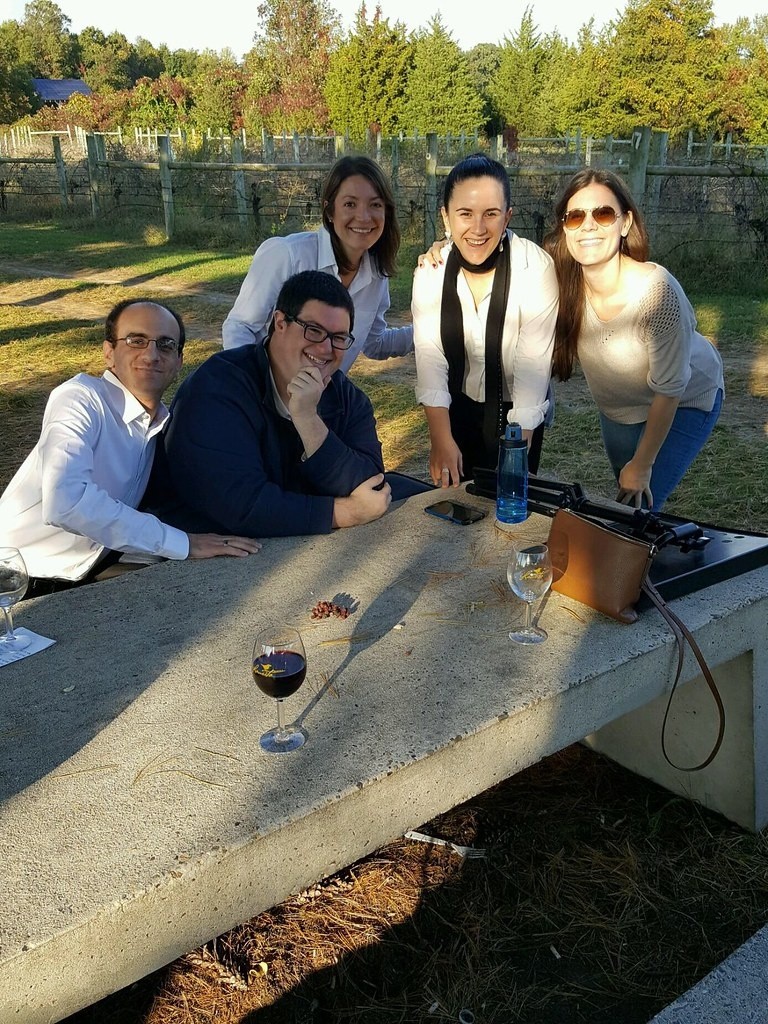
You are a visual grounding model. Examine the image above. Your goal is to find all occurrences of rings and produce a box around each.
[224,540,228,546]
[442,468,449,472]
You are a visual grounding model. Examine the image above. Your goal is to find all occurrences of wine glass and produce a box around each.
[507,540,552,645]
[0,547,32,653]
[251,627,307,752]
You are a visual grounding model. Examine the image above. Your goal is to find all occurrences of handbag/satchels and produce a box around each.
[538,507,657,625]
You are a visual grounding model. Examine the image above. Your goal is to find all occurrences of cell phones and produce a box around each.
[425,500,485,526]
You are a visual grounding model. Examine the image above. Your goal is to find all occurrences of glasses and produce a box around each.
[110,333,184,355]
[560,205,624,232]
[282,311,356,351]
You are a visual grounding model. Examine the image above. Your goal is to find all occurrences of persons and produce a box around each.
[1,296,262,605]
[220,156,414,375]
[139,268,392,535]
[411,151,559,488]
[419,168,725,511]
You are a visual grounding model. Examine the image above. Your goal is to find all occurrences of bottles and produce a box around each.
[497,422,528,523]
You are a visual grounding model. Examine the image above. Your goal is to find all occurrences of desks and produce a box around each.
[0,478,768,1024]
